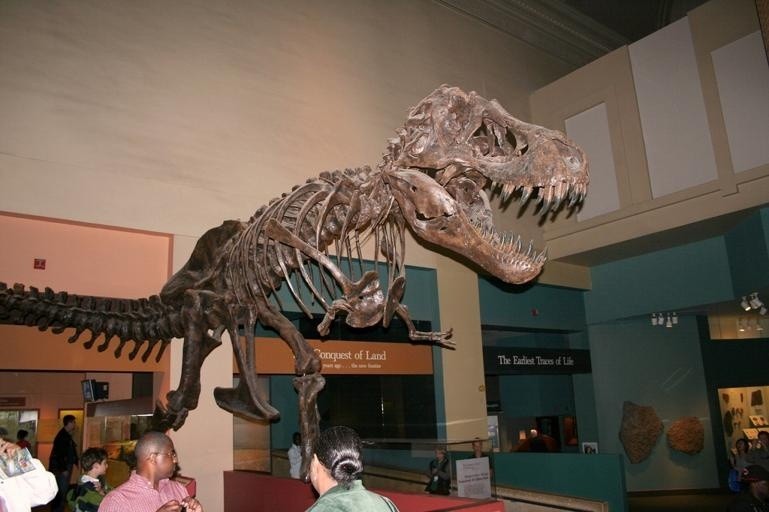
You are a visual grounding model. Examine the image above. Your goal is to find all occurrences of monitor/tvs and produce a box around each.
[81,379,97,402]
[96,382,109,401]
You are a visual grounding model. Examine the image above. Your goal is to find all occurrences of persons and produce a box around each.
[304,425,400,512]
[470,437,493,481]
[424,445,451,495]
[97,431,203,512]
[527,429,550,453]
[70,447,109,512]
[0,415,78,512]
[728,431,769,512]
[287,432,302,480]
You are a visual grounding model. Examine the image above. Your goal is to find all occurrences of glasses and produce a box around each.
[149,450,178,459]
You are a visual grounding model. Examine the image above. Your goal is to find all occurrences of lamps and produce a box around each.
[738,292,768,316]
[651,310,679,329]
